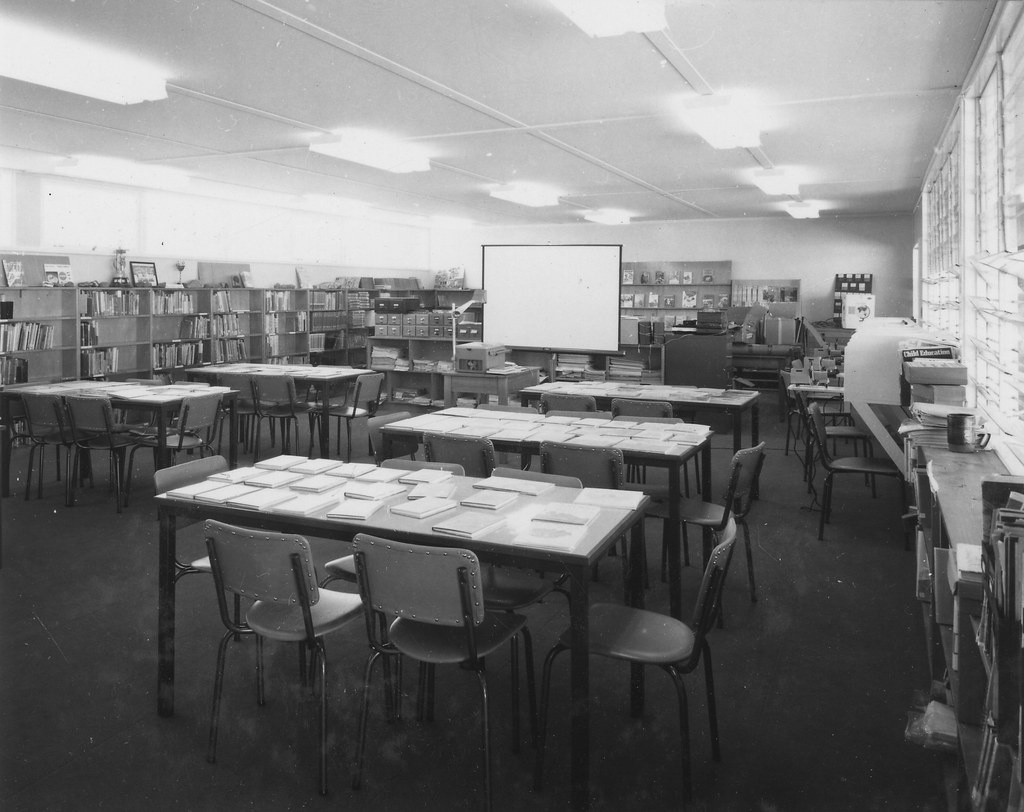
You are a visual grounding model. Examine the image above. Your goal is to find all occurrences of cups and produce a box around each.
[947,414,975,453]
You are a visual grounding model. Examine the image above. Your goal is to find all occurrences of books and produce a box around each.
[347,291,369,348]
[108,389,185,403]
[167,455,645,554]
[3,259,27,287]
[384,407,711,454]
[212,291,248,362]
[621,270,729,326]
[0,321,54,384]
[524,381,727,403]
[153,289,207,385]
[310,290,346,351]
[80,290,139,376]
[265,290,307,364]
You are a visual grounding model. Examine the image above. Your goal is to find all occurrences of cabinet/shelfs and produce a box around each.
[365,336,665,422]
[0,286,482,448]
[731,279,801,346]
[911,446,1024,812]
[619,260,731,345]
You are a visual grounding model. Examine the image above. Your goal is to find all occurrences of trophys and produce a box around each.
[109,249,132,287]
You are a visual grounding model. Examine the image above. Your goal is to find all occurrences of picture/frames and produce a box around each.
[130,260,159,287]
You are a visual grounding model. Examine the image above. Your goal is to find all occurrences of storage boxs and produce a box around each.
[767,318,796,345]
[638,322,665,370]
[904,359,967,385]
[697,311,727,334]
[912,384,965,408]
[842,293,875,330]
[375,298,483,339]
[455,341,505,372]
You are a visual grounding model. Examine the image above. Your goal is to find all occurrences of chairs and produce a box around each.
[778,348,913,551]
[0,373,764,812]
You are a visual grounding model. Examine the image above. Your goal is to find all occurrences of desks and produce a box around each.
[442,366,541,464]
[517,380,760,523]
[804,323,855,354]
[185,363,376,458]
[379,407,712,621]
[156,456,652,812]
[0,380,241,504]
